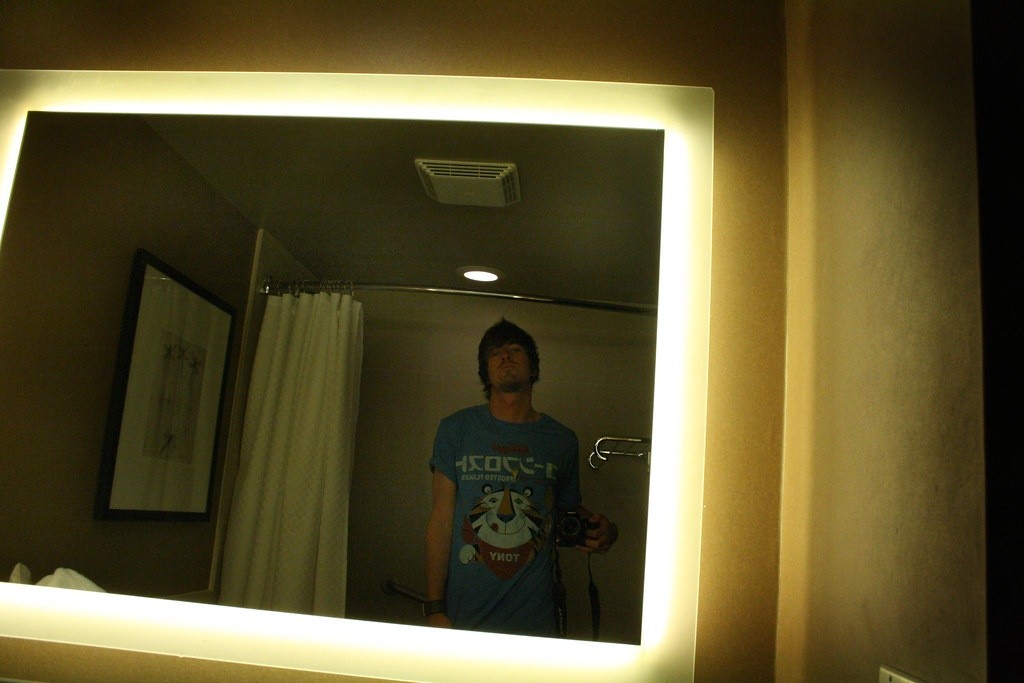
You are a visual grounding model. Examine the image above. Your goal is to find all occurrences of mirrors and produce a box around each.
[0,69,718,683]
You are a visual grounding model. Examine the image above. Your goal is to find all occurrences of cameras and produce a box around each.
[551,513,600,547]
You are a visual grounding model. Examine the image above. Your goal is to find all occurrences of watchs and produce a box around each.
[423,599,446,616]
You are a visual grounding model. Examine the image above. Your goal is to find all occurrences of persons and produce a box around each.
[426,317,621,642]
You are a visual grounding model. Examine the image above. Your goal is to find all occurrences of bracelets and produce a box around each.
[610,523,618,541]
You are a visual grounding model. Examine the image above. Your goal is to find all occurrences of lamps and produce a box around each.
[455,266,506,282]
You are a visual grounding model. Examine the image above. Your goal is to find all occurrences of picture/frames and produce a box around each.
[95,248,238,523]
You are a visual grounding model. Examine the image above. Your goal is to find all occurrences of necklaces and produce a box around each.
[488,408,535,482]
